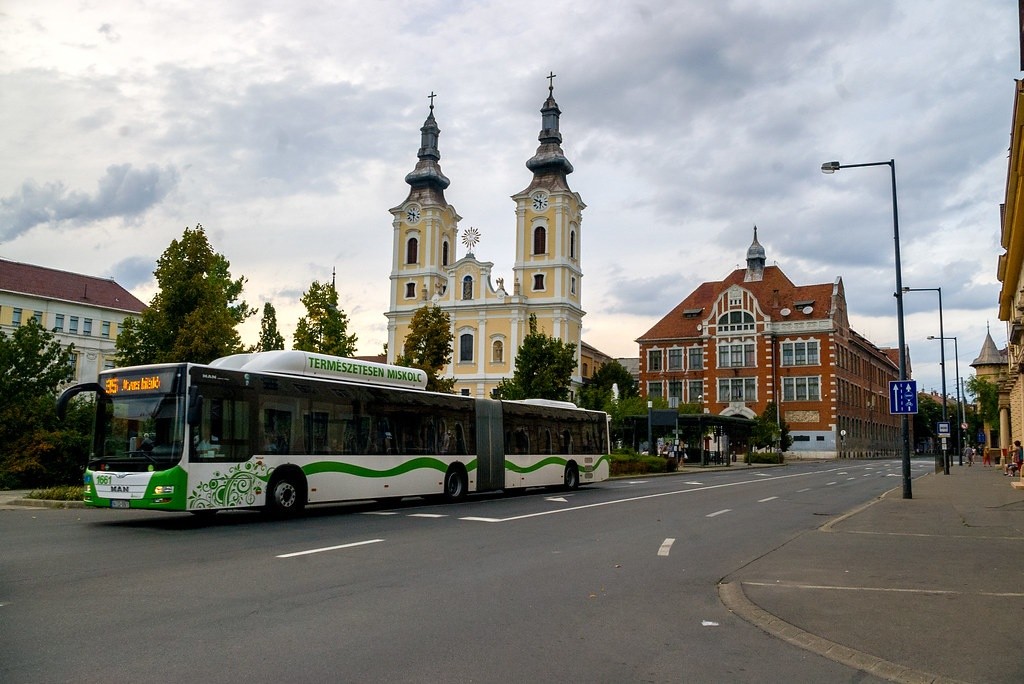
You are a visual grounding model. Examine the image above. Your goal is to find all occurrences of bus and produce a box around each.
[53,349,612,520]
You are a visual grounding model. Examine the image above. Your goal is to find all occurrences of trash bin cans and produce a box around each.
[995,456,1000,464]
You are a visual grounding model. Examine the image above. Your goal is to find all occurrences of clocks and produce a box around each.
[532,193,548,210]
[406,206,420,223]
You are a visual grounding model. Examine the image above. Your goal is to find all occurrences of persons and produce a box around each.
[193,417,221,458]
[964,444,973,466]
[1007,445,1023,476]
[971,446,977,464]
[983,442,993,467]
[1003,440,1023,477]
[140,433,152,444]
[661,438,684,469]
[256,430,343,453]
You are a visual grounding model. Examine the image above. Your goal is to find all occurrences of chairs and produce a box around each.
[704,450,729,464]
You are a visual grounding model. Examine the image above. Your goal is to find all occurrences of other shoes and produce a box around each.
[1003,474,1008,476]
[1012,474,1015,476]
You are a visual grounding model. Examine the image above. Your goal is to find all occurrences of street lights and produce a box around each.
[817,158,912,499]
[903,287,950,475]
[927,335,962,467]
